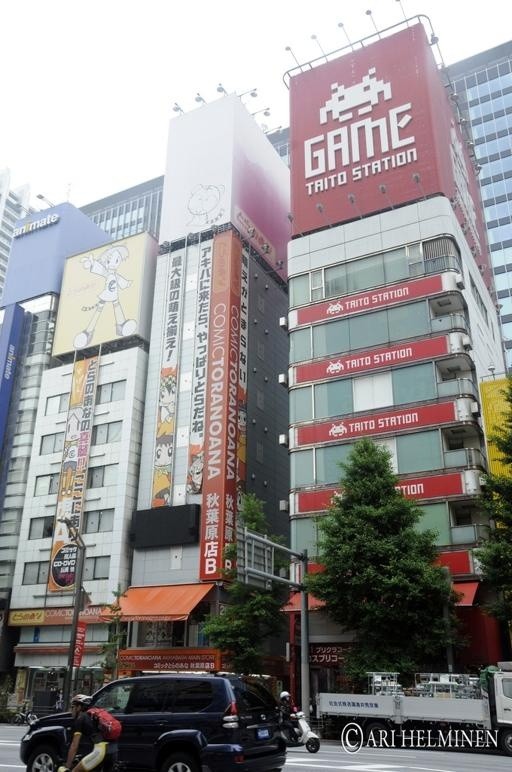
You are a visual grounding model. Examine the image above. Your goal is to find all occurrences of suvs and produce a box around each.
[19,669,289,772]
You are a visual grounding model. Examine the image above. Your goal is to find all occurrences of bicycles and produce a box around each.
[10,700,38,727]
[55,755,131,772]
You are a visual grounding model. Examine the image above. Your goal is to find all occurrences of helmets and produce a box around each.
[72,693,94,706]
[279,691,292,700]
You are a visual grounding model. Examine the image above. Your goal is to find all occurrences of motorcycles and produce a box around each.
[278,710,322,754]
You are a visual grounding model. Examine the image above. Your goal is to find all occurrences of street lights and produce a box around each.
[54,515,88,714]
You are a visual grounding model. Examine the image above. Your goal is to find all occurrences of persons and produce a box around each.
[276,691,299,743]
[58,693,123,770]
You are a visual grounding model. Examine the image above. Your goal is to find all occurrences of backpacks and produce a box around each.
[85,705,123,741]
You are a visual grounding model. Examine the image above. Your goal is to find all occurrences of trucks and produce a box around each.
[314,660,512,759]
